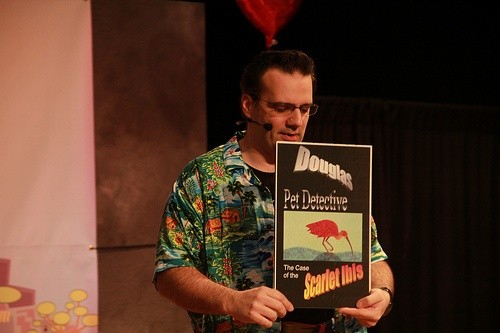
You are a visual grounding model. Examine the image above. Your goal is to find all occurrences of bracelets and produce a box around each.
[380,287,393,317]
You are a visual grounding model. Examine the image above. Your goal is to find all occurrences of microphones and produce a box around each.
[245,118,273,131]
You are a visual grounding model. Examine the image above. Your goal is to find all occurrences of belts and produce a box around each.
[281,323,336,333]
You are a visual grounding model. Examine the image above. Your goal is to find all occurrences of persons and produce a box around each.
[152,50,394,333]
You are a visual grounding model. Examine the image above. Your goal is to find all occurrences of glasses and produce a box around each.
[256,97,319,119]
